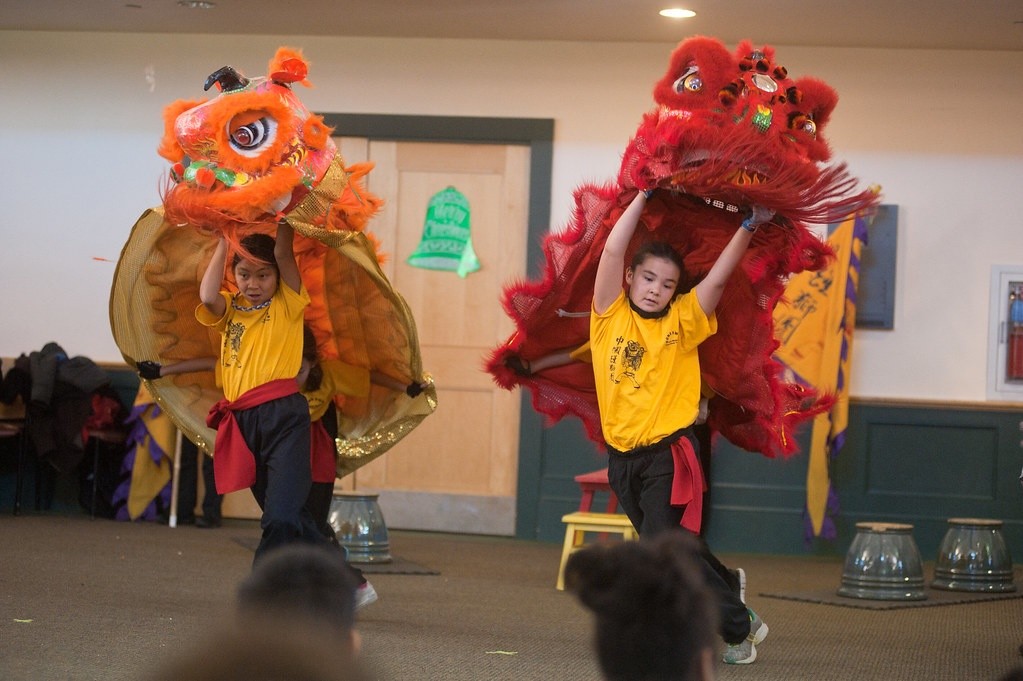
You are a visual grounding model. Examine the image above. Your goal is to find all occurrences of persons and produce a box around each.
[505,338,714,542]
[135,325,425,561]
[563,542,716,681]
[591,189,776,666]
[195,217,378,626]
[238,545,360,681]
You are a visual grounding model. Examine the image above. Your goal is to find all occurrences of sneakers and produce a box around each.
[722,608,768,666]
[728,567,747,604]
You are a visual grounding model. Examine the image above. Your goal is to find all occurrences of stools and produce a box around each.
[573,468,619,545]
[556,511,640,591]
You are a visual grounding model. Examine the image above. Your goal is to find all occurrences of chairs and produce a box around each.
[85,405,135,520]
[0,395,29,517]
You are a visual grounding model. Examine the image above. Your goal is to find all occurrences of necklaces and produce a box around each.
[232,291,272,312]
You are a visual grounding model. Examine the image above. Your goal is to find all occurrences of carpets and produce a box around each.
[231,535,441,576]
[759,582,1023,611]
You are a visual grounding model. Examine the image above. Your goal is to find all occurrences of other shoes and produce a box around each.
[197,514,225,526]
[164,510,195,523]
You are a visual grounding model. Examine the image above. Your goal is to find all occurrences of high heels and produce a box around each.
[351,581,378,610]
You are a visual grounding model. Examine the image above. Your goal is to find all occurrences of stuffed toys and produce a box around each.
[484,36,877,460]
[108,46,439,478]
